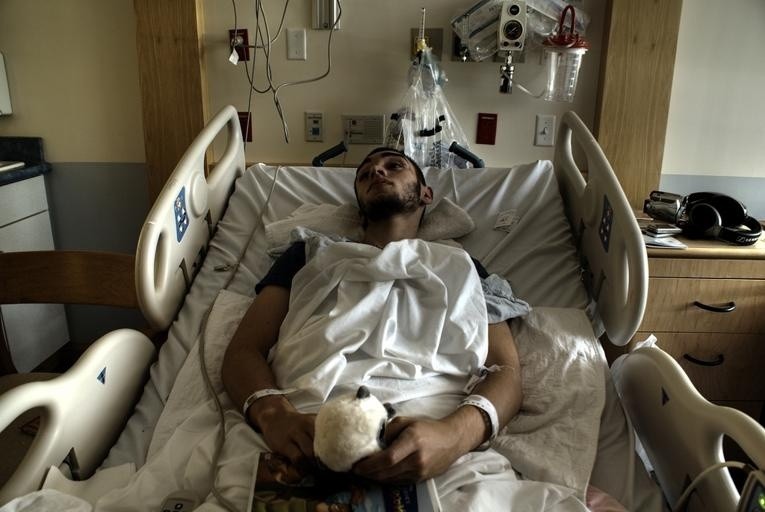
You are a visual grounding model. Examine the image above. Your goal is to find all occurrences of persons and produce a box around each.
[217,146,524,489]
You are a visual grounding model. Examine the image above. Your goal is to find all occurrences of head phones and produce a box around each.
[682,191,763,247]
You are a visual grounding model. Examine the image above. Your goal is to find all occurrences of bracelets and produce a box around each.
[457,393,500,453]
[240,389,288,415]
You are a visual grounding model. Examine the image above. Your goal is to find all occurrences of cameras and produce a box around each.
[643,190,685,227]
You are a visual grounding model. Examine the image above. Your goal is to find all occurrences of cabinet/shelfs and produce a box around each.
[625,210,765,404]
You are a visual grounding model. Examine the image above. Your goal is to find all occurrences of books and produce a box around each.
[245,450,443,511]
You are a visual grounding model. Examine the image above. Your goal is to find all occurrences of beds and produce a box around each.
[0,110,765,512]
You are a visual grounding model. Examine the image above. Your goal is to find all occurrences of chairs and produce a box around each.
[1,249,158,506]
